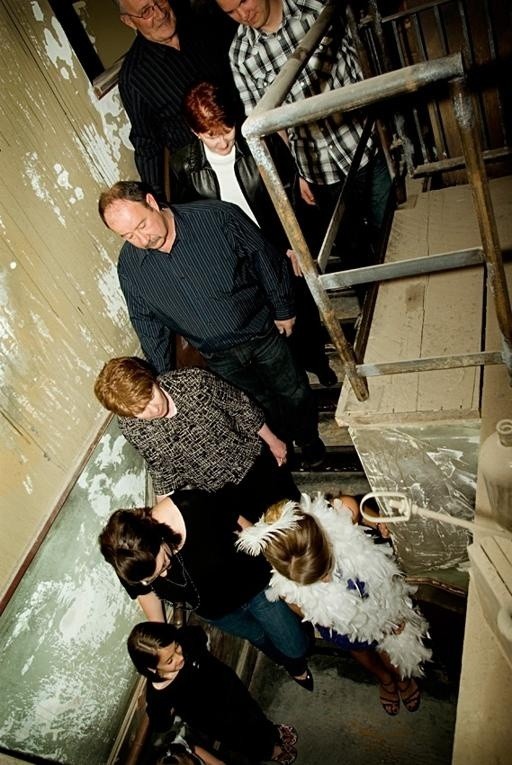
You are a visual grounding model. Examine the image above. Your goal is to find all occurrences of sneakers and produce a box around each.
[303,439,328,466]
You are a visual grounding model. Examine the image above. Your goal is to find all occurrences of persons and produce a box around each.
[127,621,299,765]
[174,83,340,386]
[234,492,450,717]
[99,486,347,692]
[117,0,235,205]
[144,741,222,765]
[94,357,301,525]
[98,180,327,474]
[219,0,396,316]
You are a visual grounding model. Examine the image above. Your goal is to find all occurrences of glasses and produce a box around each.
[139,545,172,585]
[128,0,166,19]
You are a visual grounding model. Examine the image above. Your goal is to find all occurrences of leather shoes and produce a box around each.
[270,724,297,764]
[316,366,337,387]
[288,665,313,692]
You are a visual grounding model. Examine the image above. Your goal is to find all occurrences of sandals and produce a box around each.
[379,675,420,715]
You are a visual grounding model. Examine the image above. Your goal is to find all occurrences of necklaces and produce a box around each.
[149,552,201,615]
